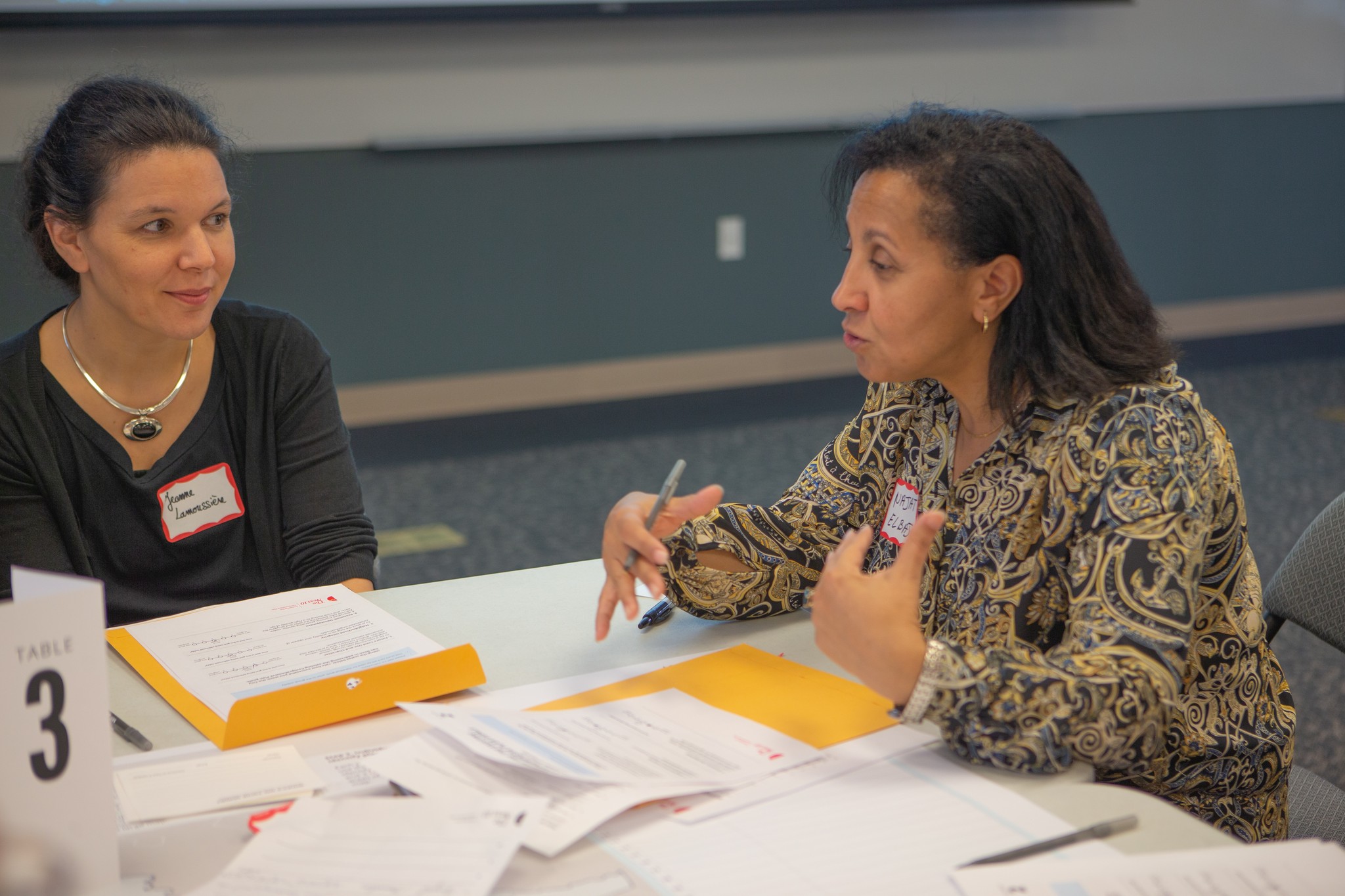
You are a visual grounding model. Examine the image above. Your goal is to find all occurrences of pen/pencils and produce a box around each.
[957,815,1142,868]
[637,596,675,630]
[622,460,686,570]
[109,713,153,752]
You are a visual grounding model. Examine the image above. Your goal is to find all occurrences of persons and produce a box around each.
[0,78,378,632]
[594,103,1294,846]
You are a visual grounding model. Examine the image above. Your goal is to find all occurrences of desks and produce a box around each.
[108,551,1248,896]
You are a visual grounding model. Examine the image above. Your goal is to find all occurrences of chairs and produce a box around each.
[1262,485,1345,849]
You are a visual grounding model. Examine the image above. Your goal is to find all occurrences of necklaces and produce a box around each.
[62,297,193,440]
[960,390,1032,438]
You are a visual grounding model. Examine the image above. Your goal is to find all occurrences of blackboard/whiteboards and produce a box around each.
[1,4,1343,431]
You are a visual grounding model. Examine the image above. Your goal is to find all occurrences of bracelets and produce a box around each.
[888,637,943,728]
